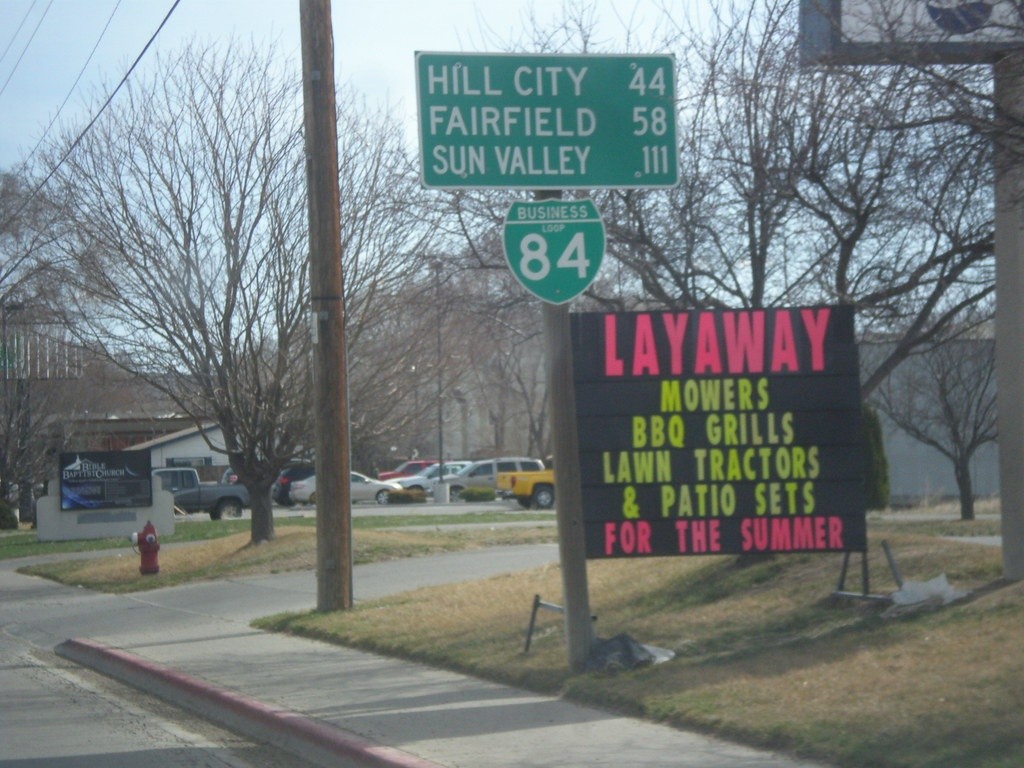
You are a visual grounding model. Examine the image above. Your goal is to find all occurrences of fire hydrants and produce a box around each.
[125,520,163,576]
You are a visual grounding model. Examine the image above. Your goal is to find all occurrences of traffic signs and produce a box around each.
[412,49,679,190]
[502,197,606,308]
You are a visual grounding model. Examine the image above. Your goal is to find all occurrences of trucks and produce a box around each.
[494,468,557,509]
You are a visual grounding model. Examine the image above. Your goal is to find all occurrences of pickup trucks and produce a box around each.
[432,456,545,505]
[151,467,251,522]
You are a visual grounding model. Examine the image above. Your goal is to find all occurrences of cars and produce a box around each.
[288,468,404,508]
[384,459,474,500]
[269,461,316,507]
[378,459,441,480]
